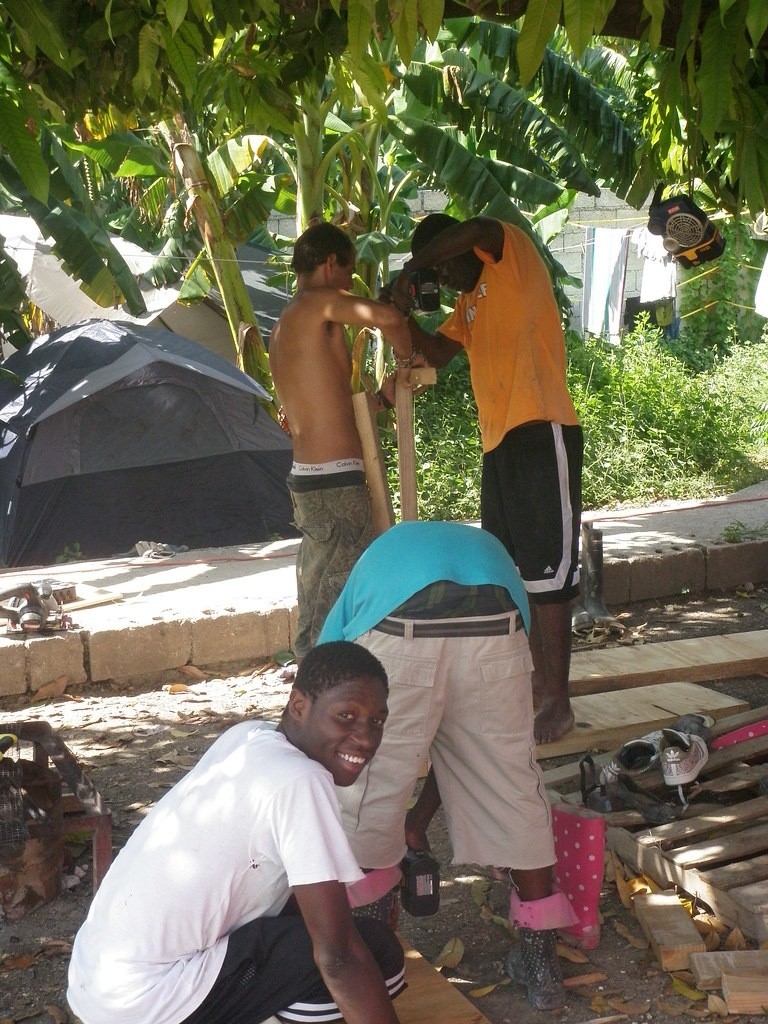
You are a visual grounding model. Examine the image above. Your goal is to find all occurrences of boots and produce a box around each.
[546,787,607,950]
[345,865,405,933]
[505,885,578,1009]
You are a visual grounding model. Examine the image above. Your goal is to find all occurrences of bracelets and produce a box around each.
[389,346,416,368]
[377,390,392,410]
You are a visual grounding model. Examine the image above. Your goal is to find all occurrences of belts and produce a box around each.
[374,615,524,637]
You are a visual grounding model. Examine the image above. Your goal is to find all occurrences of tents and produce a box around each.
[0,215,293,571]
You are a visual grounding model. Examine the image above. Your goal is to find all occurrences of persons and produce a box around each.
[267,223,416,667]
[66,642,408,1024]
[314,522,566,1011]
[380,214,585,745]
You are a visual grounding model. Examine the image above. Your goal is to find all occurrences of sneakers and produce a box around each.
[613,728,709,789]
